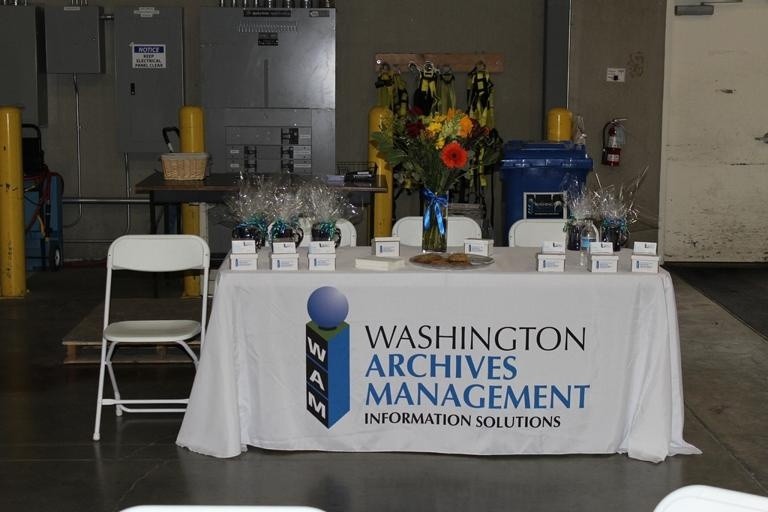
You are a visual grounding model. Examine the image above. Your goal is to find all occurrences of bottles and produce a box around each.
[567,225,582,250]
[580,218,599,268]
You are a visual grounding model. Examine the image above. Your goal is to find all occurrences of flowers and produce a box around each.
[372,113,504,250]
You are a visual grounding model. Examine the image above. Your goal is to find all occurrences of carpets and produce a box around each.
[676,267,768,341]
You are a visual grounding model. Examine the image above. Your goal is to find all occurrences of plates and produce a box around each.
[408,252,494,270]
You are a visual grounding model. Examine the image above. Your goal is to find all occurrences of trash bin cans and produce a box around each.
[499,140,593,247]
[18,174,65,273]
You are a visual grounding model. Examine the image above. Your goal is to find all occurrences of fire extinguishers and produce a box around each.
[602,118,628,168]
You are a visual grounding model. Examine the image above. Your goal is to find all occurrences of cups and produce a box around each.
[602,227,630,251]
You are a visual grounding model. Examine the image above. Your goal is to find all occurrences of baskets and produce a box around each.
[161,152,209,180]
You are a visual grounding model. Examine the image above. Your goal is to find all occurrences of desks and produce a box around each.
[177,246,702,465]
[134,173,388,296]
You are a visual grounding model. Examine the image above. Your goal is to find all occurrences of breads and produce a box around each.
[449,253,468,262]
[416,254,441,264]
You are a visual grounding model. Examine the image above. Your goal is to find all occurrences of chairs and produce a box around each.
[509,218,568,248]
[92,235,211,442]
[391,217,482,246]
[265,217,357,247]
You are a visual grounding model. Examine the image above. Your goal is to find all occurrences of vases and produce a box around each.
[423,181,448,253]
